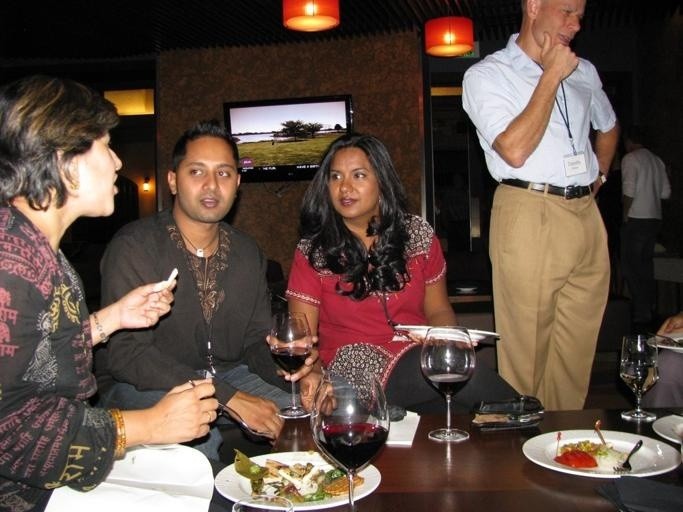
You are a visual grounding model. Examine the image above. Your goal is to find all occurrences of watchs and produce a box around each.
[596,170,607,187]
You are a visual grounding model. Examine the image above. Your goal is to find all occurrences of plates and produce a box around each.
[214,450,381,511]
[652,414,683,444]
[521,429,682,478]
[647,333,682,353]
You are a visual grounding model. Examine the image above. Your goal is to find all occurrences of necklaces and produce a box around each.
[177,226,218,256]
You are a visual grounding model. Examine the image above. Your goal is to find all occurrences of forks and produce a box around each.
[614,438,643,473]
[189,379,275,438]
[655,334,683,347]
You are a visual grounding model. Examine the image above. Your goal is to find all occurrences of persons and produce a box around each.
[0,77,218,511]
[462,0,617,411]
[657,310,683,341]
[285,134,524,414]
[620,126,670,333]
[94,123,318,443]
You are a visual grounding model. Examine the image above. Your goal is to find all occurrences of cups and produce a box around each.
[232,494,294,511]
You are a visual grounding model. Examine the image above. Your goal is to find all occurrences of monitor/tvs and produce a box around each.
[220,93,353,184]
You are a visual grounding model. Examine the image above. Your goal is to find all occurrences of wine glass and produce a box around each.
[309,372,391,512]
[419,326,476,443]
[269,312,313,419]
[620,334,660,422]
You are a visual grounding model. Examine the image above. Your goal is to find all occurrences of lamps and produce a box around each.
[281,0,340,33]
[143,176,149,191]
[423,7,474,58]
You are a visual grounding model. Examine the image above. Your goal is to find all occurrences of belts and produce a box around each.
[501,179,595,200]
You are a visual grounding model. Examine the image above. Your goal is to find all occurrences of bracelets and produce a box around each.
[111,408,125,459]
[92,312,107,344]
